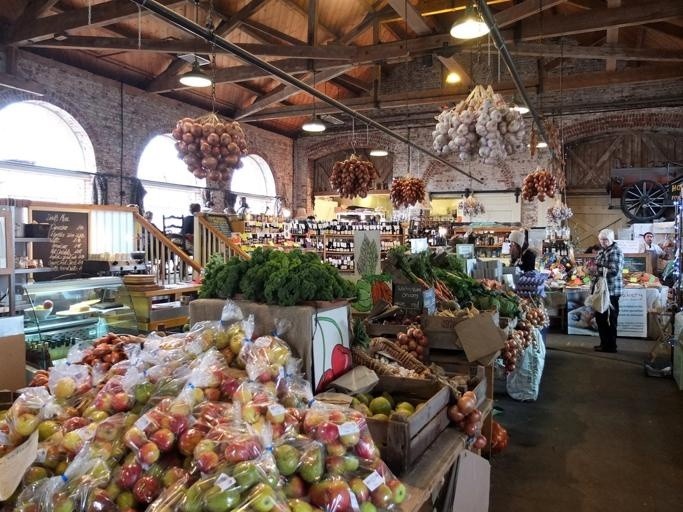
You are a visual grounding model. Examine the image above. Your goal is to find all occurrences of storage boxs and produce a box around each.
[313,303,511,472]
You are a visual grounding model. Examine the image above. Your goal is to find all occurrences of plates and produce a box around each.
[123,274,155,284]
[55,308,99,316]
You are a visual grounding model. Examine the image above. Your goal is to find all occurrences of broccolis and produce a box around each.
[196,246,359,307]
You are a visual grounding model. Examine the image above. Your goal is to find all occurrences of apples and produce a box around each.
[44,302,52,308]
[0,326,425,512]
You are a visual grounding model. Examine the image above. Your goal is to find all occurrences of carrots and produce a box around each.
[370,279,394,309]
[416,277,454,305]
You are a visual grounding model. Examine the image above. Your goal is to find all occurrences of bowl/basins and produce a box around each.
[23,309,51,319]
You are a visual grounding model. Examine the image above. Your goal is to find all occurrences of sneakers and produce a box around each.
[594,344,618,354]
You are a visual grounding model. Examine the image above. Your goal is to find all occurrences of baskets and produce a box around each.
[353,336,427,378]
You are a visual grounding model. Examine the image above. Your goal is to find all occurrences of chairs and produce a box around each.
[158,214,188,281]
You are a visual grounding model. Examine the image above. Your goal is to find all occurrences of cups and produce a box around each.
[179,296,190,305]
[18,256,43,269]
[103,253,130,261]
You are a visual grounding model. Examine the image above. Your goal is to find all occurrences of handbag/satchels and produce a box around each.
[590,277,611,313]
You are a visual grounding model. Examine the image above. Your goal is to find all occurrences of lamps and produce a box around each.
[300,67,326,135]
[450,0,490,41]
[176,0,211,88]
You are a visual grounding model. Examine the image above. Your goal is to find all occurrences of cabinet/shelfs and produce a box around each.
[10,278,143,386]
[229,216,654,276]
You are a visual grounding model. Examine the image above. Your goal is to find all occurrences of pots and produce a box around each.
[23,224,50,238]
[130,252,146,259]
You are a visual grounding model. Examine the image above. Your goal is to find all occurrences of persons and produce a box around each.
[509,229,535,269]
[641,233,663,256]
[588,229,623,352]
[584,244,602,254]
[181,203,201,256]
[143,212,161,232]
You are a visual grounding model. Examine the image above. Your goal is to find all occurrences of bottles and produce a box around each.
[474,233,509,258]
[25,333,77,370]
[542,233,567,256]
[244,214,402,274]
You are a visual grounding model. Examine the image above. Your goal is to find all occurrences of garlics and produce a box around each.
[458,196,485,217]
[546,203,571,225]
[432,85,526,160]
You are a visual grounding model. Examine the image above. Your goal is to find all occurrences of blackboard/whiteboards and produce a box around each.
[204,213,232,244]
[26,205,92,282]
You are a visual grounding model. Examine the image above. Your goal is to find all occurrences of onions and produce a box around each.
[390,174,425,207]
[500,309,545,372]
[329,159,377,199]
[519,171,558,202]
[171,118,249,181]
[448,390,509,453]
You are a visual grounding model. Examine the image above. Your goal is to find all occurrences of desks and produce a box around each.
[562,282,663,337]
